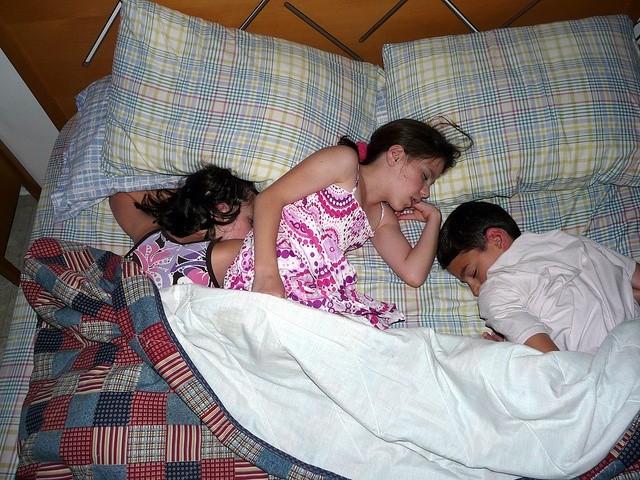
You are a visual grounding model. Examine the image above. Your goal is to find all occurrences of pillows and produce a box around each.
[382,15,639,207]
[52,75,260,226]
[101,1,378,194]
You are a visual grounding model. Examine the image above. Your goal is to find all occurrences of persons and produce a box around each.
[109,162,259,293]
[222,113,474,331]
[435,202,639,354]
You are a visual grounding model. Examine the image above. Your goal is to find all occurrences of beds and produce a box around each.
[1,1,640,479]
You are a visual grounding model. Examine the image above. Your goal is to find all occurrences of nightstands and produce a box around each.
[0,139,44,288]
